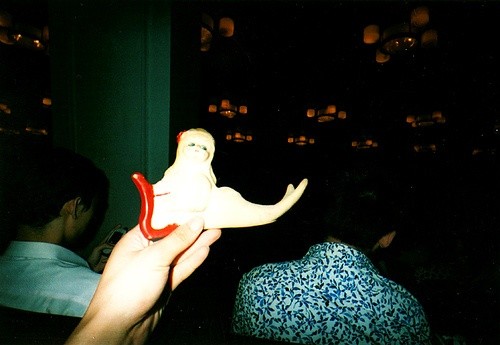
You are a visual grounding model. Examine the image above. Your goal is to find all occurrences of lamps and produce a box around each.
[198,5,446,150]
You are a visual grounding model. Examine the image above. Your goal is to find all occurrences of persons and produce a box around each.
[131,127,309,242]
[64,218,221,345]
[230,147,431,345]
[1,149,111,318]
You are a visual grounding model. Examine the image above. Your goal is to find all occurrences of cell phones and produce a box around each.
[102,228,127,255]
